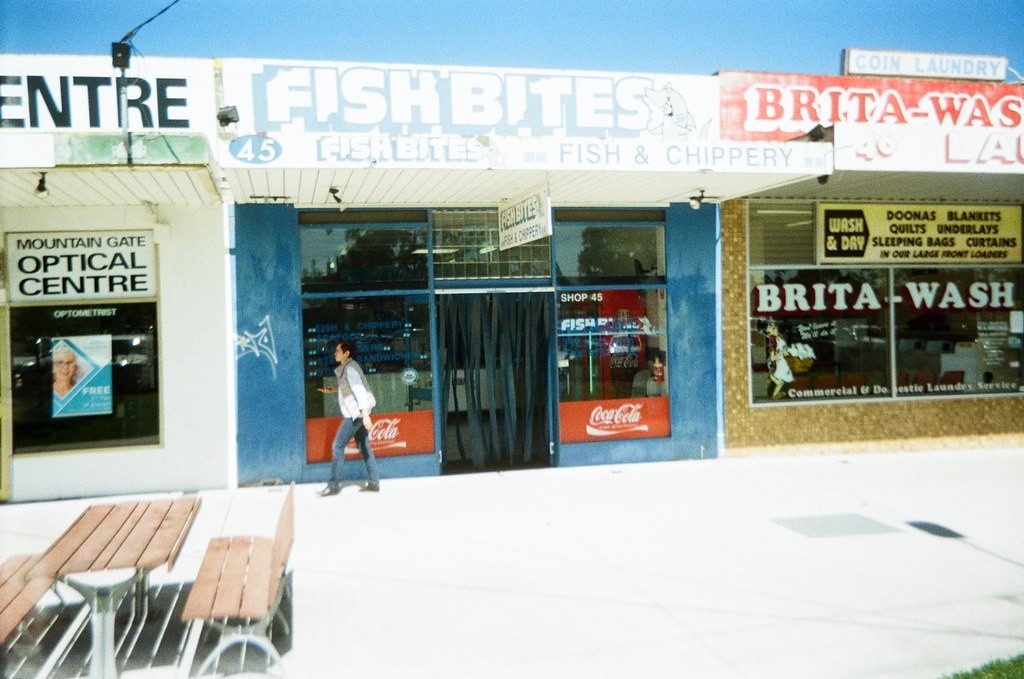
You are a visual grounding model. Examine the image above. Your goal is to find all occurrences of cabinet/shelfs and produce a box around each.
[846,343,976,384]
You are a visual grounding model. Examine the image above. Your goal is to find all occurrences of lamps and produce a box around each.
[217,105,240,128]
[35,172,49,198]
[327,188,349,213]
[689,190,706,210]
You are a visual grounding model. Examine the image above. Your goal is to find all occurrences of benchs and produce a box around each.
[184,480,296,678]
[0,553,68,647]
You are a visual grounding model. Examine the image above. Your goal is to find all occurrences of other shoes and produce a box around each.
[365,481,379,491]
[320,485,337,496]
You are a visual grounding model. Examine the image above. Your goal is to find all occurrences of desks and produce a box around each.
[22,495,202,679]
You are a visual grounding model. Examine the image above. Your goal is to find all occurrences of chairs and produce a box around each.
[788,370,966,398]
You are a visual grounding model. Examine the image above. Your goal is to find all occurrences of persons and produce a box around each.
[53,346,77,398]
[314,341,380,498]
